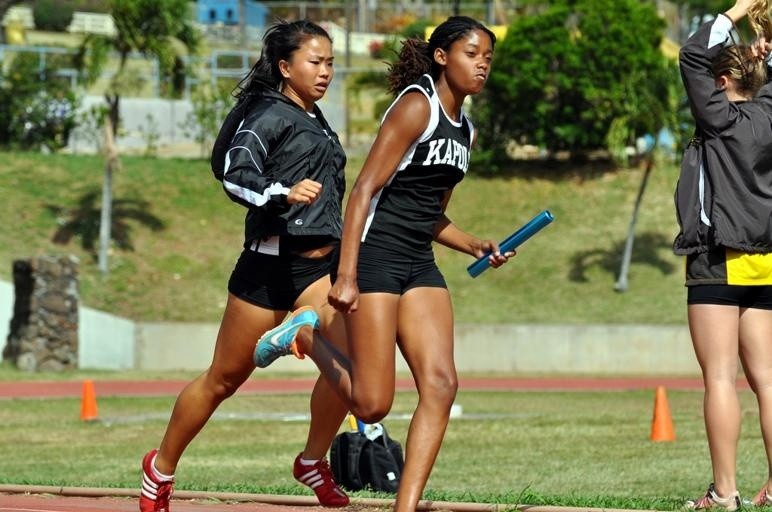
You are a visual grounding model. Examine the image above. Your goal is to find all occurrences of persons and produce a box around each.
[251,15,516,510]
[136,18,350,511]
[671,1,771,511]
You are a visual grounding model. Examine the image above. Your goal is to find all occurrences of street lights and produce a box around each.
[649,385,677,443]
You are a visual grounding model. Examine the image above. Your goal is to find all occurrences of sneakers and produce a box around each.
[293,448,350,507]
[138,448,173,512]
[253,303,320,369]
[741,486,772,508]
[684,484,741,511]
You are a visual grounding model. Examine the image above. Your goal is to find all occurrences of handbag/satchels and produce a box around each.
[329,424,407,494]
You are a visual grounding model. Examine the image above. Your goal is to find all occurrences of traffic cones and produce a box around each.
[80,382,99,420]
